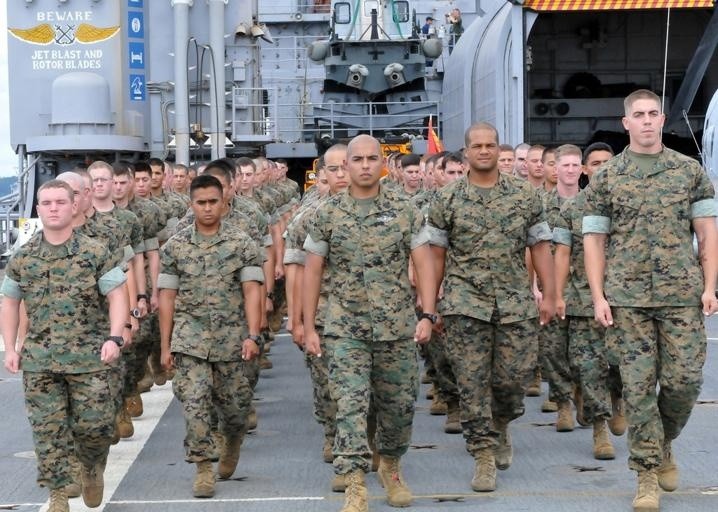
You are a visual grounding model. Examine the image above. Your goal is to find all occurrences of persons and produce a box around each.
[445,8,462,57]
[421,17,435,67]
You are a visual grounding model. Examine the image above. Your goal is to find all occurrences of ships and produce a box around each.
[8,0,717,511]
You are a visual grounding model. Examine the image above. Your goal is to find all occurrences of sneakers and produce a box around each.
[331,473,348,493]
[64,475,83,498]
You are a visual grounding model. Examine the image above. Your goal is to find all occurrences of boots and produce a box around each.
[219,301,290,480]
[189,461,219,500]
[471,447,498,493]
[338,468,371,512]
[632,469,663,512]
[78,462,107,508]
[106,340,173,446]
[656,437,681,493]
[421,371,626,470]
[45,484,71,512]
[375,453,415,508]
[321,433,338,462]
